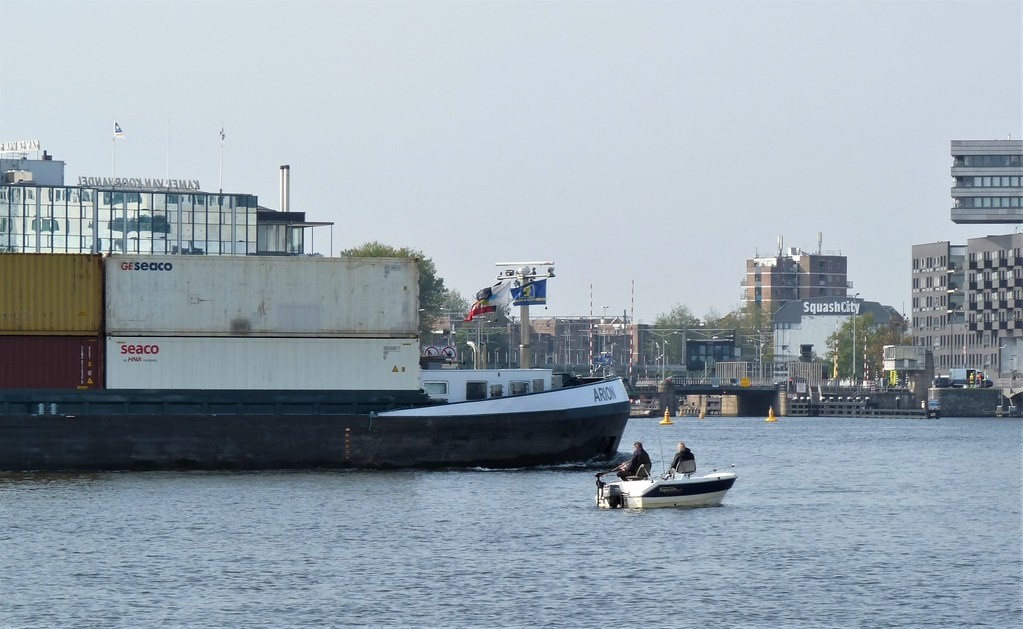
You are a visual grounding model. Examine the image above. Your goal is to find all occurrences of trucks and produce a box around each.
[948,368,993,388]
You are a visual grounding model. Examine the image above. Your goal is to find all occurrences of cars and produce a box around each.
[934,376,949,388]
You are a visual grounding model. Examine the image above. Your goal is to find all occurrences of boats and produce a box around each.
[420,368,555,403]
[595,472,737,509]
[1,153,631,476]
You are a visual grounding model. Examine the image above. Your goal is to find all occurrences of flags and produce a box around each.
[115,123,125,138]
[463,300,496,321]
[510,278,546,306]
[473,280,510,305]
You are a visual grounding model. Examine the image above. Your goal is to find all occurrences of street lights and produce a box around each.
[477,316,486,369]
[786,347,792,392]
[600,304,609,357]
[480,320,492,368]
[745,335,774,380]
[560,319,571,364]
[655,341,661,373]
[664,339,670,369]
[674,331,684,365]
[688,328,729,384]
[836,293,860,377]
[642,328,684,381]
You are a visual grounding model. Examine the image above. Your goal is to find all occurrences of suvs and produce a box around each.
[774,377,806,392]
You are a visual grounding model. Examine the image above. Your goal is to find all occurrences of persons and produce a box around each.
[616,442,651,482]
[671,442,694,477]
[977,373,981,383]
[970,373,974,384]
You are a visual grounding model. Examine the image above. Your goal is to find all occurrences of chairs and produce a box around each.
[625,462,651,481]
[676,457,696,478]
